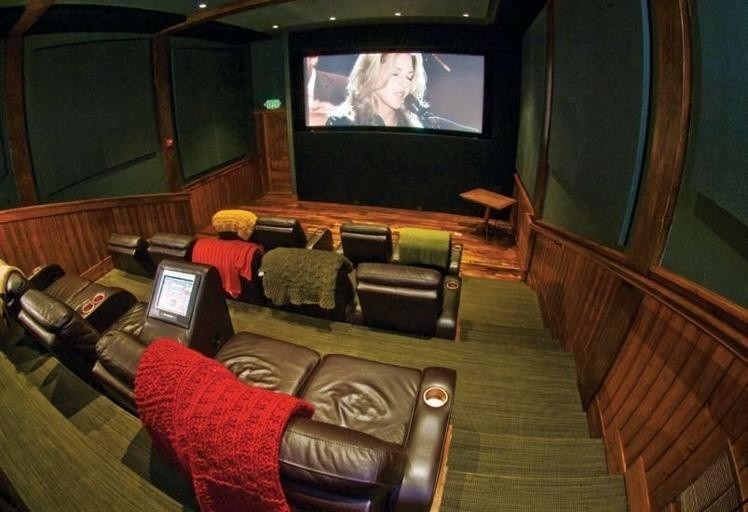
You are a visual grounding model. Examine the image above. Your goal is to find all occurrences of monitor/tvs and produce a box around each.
[149,266,200,329]
[298,49,491,140]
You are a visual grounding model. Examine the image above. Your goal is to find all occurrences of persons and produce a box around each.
[325,53,428,129]
[305,56,349,115]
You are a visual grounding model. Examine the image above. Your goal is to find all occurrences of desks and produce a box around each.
[460,188,518,242]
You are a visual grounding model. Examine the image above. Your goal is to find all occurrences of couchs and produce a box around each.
[219,353,457,511]
[0,259,67,328]
[94,328,322,441]
[188,233,262,306]
[212,210,256,242]
[106,233,155,278]
[335,223,393,267]
[147,232,193,270]
[258,248,358,324]
[355,262,463,341]
[255,217,334,252]
[17,274,148,371]
[391,228,462,276]
